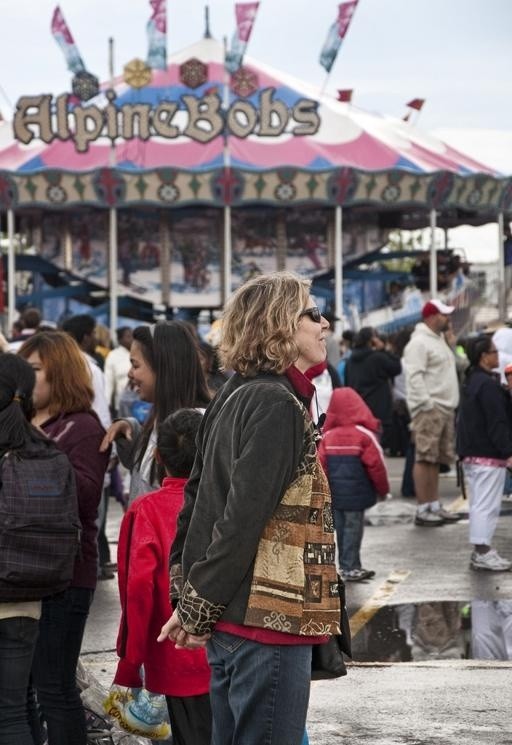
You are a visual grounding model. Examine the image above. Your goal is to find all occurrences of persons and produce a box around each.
[108,407,211,745]
[311,291,511,581]
[17,331,113,745]
[469,601,512,661]
[394,603,466,661]
[0,352,82,745]
[1,292,43,357]
[155,273,352,745]
[54,312,231,510]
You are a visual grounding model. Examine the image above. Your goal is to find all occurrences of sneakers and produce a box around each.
[339,567,375,582]
[97,559,119,580]
[470,547,512,571]
[415,505,461,527]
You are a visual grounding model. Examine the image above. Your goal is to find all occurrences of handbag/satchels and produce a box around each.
[311,578,353,681]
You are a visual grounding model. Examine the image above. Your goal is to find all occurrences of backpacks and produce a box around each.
[1,442,82,602]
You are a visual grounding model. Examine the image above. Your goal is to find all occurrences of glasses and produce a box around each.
[299,307,321,323]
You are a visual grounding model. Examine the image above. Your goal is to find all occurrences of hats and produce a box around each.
[421,299,456,319]
